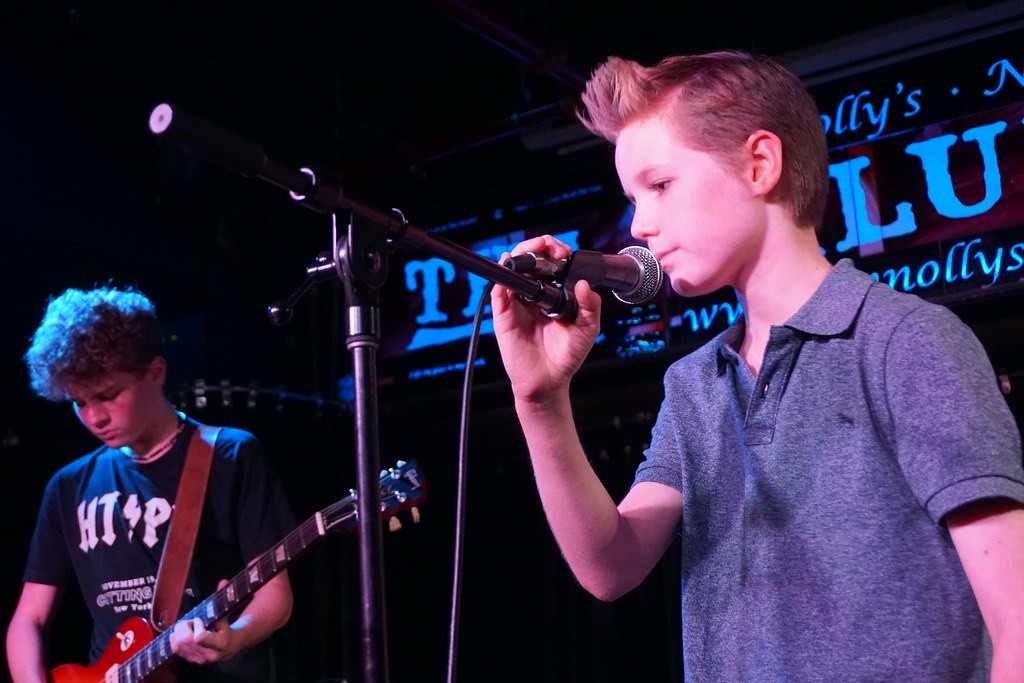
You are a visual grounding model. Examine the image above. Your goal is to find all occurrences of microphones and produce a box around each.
[507,245,664,306]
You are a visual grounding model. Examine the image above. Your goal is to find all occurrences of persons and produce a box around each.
[5,289,295,683]
[489,52,1024,683]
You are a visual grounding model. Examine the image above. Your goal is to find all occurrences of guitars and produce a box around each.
[44,454,429,683]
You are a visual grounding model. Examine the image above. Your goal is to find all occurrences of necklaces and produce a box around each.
[130,422,186,461]
